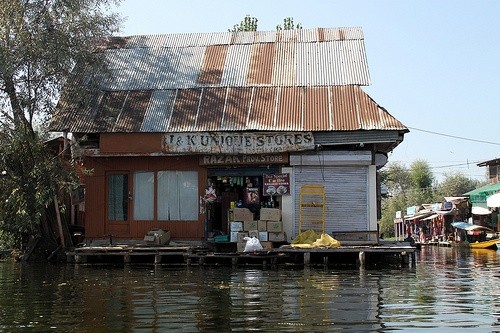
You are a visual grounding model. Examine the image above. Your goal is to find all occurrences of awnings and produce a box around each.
[463,182,500,215]
[404,207,461,221]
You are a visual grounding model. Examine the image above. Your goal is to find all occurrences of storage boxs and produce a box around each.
[258,220,266,232]
[260,207,282,221]
[268,232,285,242]
[231,221,243,232]
[233,208,254,221]
[237,243,247,252]
[259,232,268,241]
[231,232,239,242]
[144,229,172,246]
[261,242,272,248]
[266,221,282,232]
[244,220,257,231]
[249,230,258,240]
[237,232,248,243]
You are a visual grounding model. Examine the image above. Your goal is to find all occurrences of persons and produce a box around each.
[404,223,451,242]
[379,233,384,238]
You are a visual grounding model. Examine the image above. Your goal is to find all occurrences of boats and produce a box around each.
[413,222,500,248]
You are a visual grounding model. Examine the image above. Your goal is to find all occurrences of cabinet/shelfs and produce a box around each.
[299,184,326,236]
